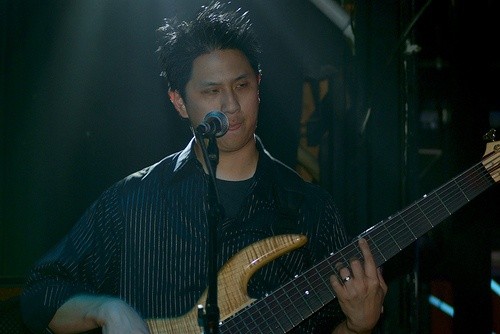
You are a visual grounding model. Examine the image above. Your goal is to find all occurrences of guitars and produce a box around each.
[143,128,500,334]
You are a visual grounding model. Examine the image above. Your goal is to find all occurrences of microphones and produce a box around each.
[195,111,229,137]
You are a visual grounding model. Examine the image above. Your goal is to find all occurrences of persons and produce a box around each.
[14,0,388,334]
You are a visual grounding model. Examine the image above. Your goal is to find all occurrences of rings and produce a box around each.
[343,276,352,284]
